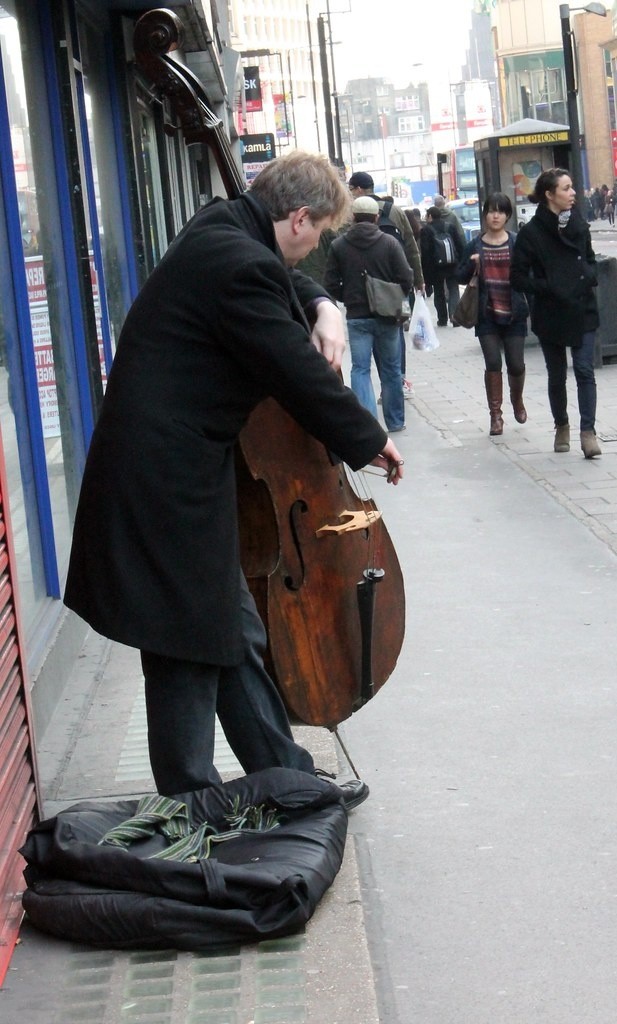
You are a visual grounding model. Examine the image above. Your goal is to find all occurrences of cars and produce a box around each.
[444,197,484,242]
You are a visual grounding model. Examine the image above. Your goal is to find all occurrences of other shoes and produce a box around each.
[389,425,406,433]
[450,318,453,323]
[437,321,447,327]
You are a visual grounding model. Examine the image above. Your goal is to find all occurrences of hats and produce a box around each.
[351,196,379,215]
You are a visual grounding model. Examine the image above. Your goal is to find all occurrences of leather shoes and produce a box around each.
[314,768,370,812]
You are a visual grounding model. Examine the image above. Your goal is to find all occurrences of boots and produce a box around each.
[508,364,527,424]
[484,370,504,435]
[580,428,602,459]
[554,424,570,452]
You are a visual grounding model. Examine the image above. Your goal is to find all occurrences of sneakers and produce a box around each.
[402,382,416,399]
[377,398,383,405]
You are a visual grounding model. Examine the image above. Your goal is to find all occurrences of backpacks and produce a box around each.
[377,200,415,270]
[425,222,461,272]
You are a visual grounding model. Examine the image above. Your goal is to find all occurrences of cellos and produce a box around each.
[130,6,409,779]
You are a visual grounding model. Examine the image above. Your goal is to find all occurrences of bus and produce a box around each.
[436,146,478,202]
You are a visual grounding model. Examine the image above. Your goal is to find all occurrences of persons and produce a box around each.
[322,168,617,458]
[63,152,407,810]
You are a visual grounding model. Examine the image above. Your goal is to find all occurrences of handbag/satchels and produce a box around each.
[365,275,412,327]
[603,203,614,214]
[453,234,480,328]
